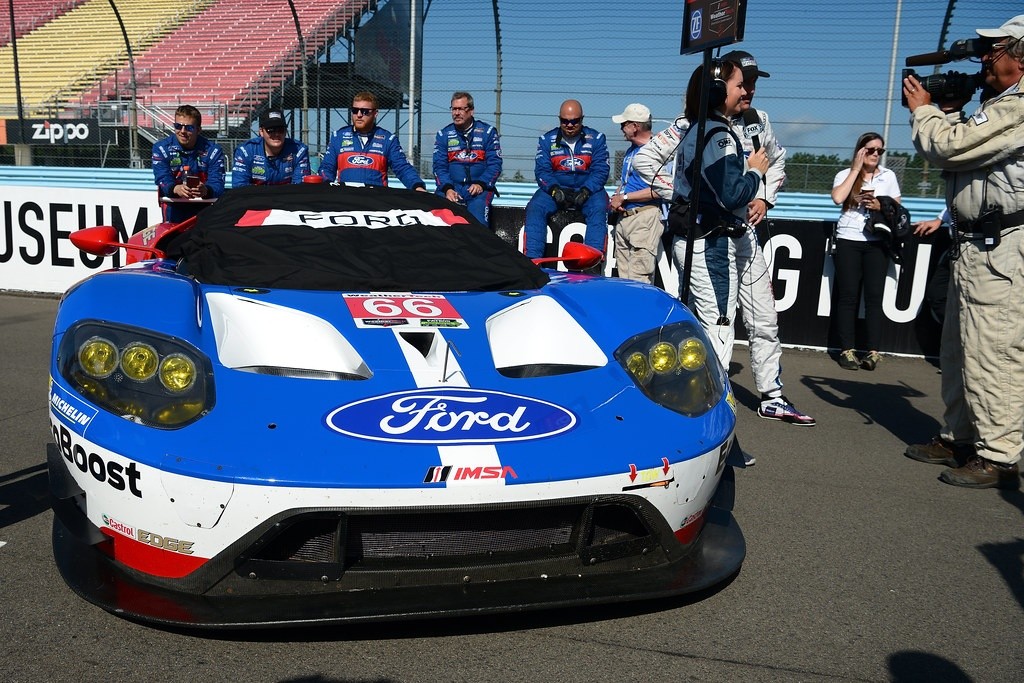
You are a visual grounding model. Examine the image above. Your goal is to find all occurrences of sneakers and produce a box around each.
[839,348,860,370]
[757,400,816,427]
[940,454,1021,491]
[861,350,883,371]
[906,433,972,469]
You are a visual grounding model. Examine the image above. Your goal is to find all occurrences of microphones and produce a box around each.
[742,108,766,184]
[905,50,955,67]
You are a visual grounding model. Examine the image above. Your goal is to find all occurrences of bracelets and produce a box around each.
[851,167,860,171]
[623,193,628,201]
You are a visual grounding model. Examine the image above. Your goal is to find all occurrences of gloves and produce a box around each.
[574,187,591,206]
[549,184,565,205]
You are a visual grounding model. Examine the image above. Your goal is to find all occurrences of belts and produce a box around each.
[958,209,1024,233]
[619,204,659,217]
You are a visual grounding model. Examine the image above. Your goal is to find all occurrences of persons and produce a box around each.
[432,91,503,229]
[607,103,666,286]
[831,132,902,370]
[903,14,1024,489]
[317,91,428,193]
[632,49,816,427]
[523,99,610,274]
[910,207,954,325]
[232,108,311,188]
[151,105,226,224]
[667,57,770,377]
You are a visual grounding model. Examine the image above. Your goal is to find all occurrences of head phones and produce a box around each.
[709,56,728,106]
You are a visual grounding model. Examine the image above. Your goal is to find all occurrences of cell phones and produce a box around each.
[186,176,199,192]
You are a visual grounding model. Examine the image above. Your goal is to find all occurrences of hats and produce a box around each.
[722,50,771,78]
[975,14,1024,41]
[612,103,651,124]
[260,107,285,128]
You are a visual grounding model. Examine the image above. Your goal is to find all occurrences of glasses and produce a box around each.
[266,127,285,133]
[558,116,584,124]
[975,41,1010,57]
[173,123,200,131]
[866,147,885,155]
[351,107,376,115]
[449,106,472,112]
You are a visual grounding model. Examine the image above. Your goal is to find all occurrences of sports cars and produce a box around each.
[46,171,750,630]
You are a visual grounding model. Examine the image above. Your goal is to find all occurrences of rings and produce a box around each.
[756,212,759,215]
[911,88,915,92]
[763,152,768,157]
